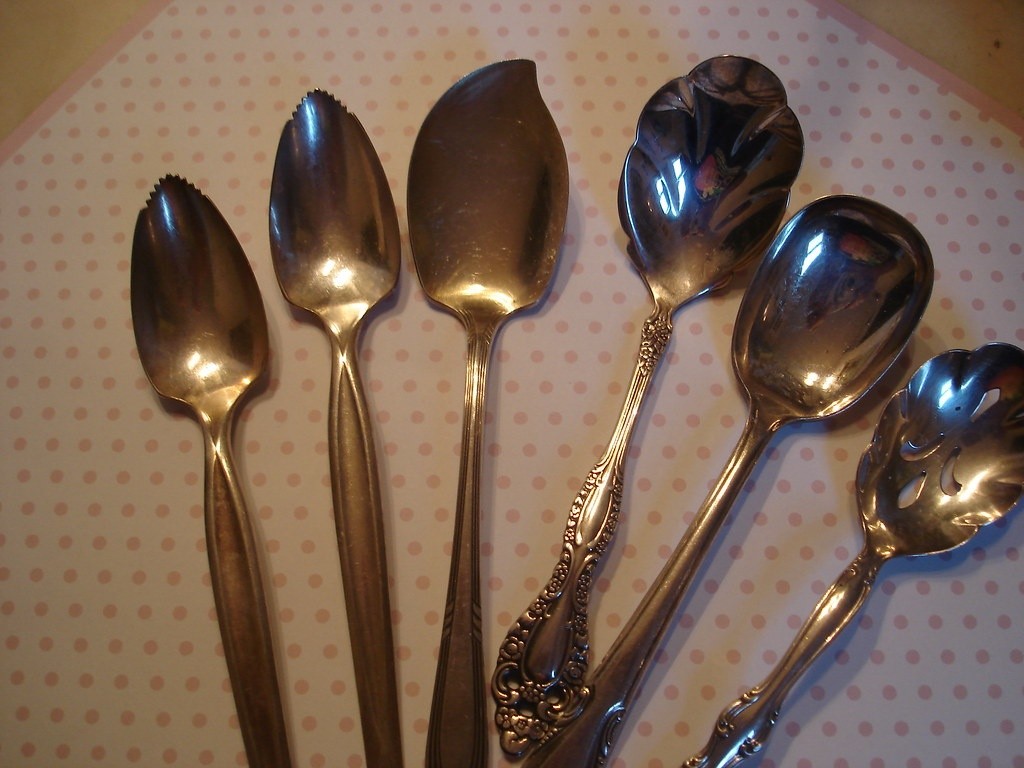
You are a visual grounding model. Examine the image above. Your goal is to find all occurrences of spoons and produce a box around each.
[681,343,1024,768]
[270,90,404,768]
[488,56,803,766]
[521,196,934,768]
[129,176,295,768]
[406,57,570,767]
